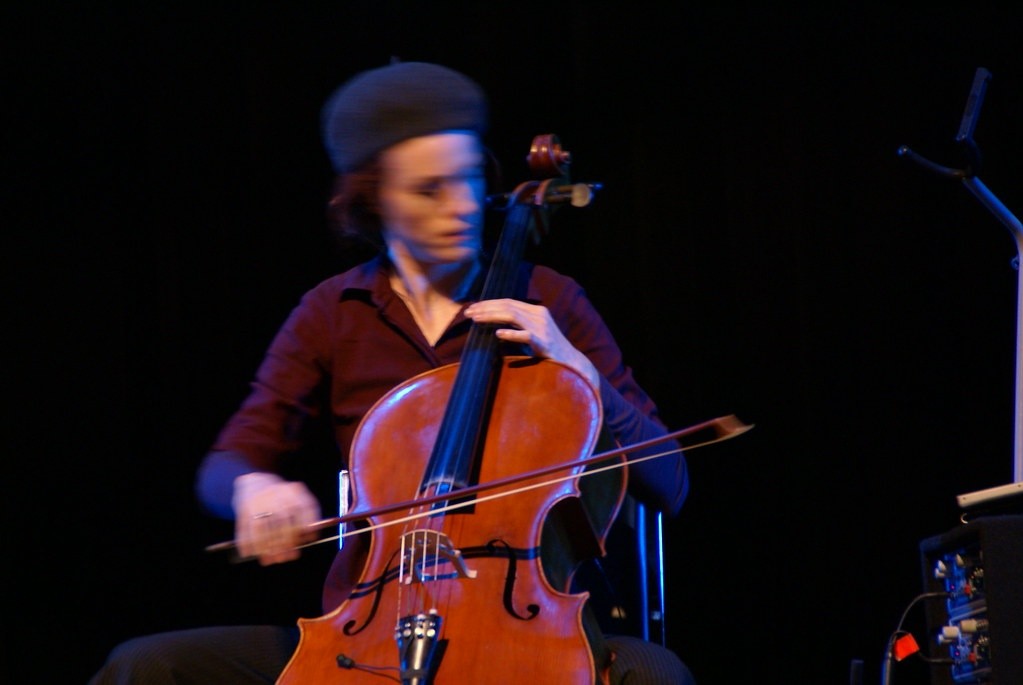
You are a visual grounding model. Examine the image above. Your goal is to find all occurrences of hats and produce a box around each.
[323,64,482,170]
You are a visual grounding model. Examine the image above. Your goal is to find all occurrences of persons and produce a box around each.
[90,59,692,685]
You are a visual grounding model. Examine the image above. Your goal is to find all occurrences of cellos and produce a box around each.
[275,131,630,685]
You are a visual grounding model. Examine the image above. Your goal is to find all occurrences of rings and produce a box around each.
[253,511,272,520]
